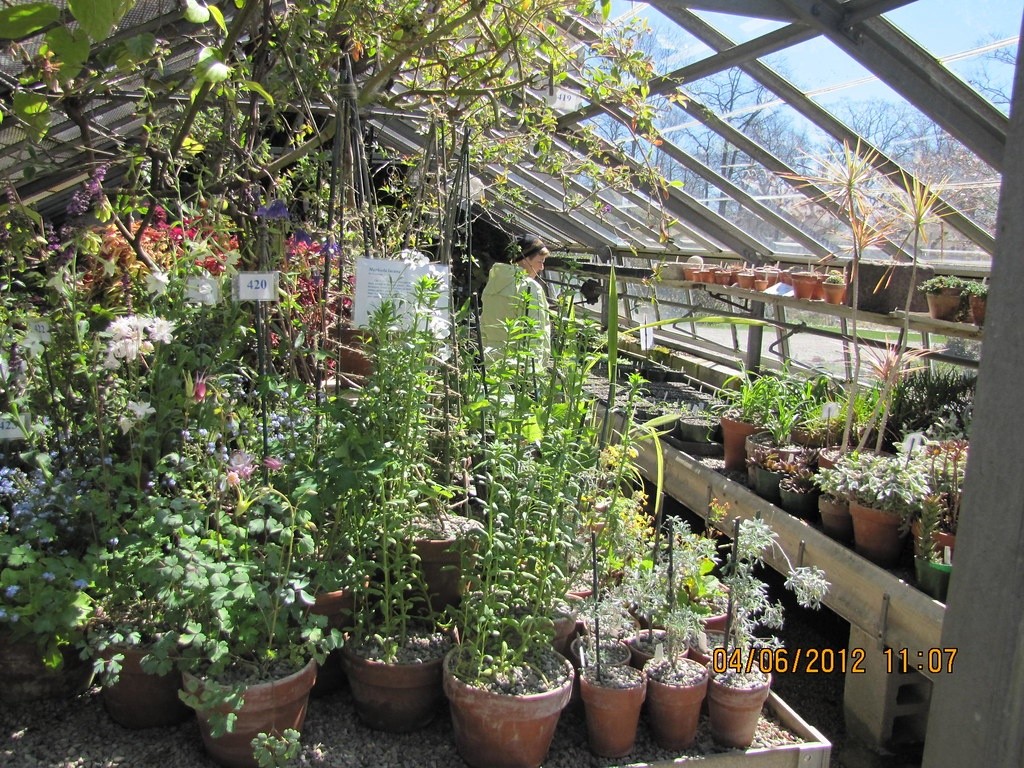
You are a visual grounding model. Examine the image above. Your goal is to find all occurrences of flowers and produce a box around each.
[0,158,357,699]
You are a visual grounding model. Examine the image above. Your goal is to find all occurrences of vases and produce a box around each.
[91,644,184,730]
[0,632,93,704]
[682,266,830,302]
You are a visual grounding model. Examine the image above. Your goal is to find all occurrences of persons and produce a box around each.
[481,234,550,457]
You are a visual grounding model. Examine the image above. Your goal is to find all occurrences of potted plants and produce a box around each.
[846,454,920,560]
[915,273,968,321]
[280,271,834,768]
[818,331,951,470]
[810,464,853,539]
[909,434,969,559]
[960,278,988,326]
[915,492,953,603]
[139,518,345,768]
[821,274,847,305]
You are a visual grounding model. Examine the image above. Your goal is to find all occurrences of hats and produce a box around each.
[510,234,545,264]
